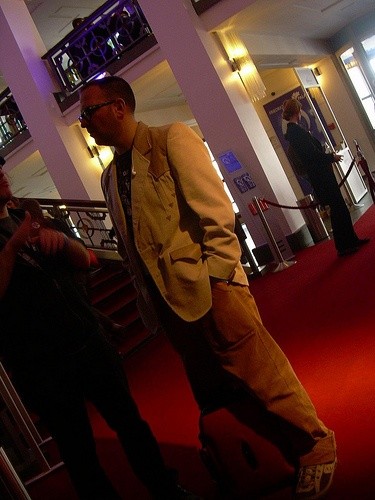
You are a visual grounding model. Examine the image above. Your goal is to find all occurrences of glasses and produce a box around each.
[79,100,116,123]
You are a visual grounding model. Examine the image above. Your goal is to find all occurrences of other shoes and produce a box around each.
[111,323,127,343]
[338,248,357,256]
[169,480,201,500]
[356,240,368,245]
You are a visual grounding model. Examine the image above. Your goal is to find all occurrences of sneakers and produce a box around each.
[296,459,337,500]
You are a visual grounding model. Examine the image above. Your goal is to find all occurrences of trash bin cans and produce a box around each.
[296,194,329,246]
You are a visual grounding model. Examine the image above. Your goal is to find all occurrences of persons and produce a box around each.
[0,167,184,499]
[282,98,369,257]
[77,74,336,500]
[68,11,140,78]
